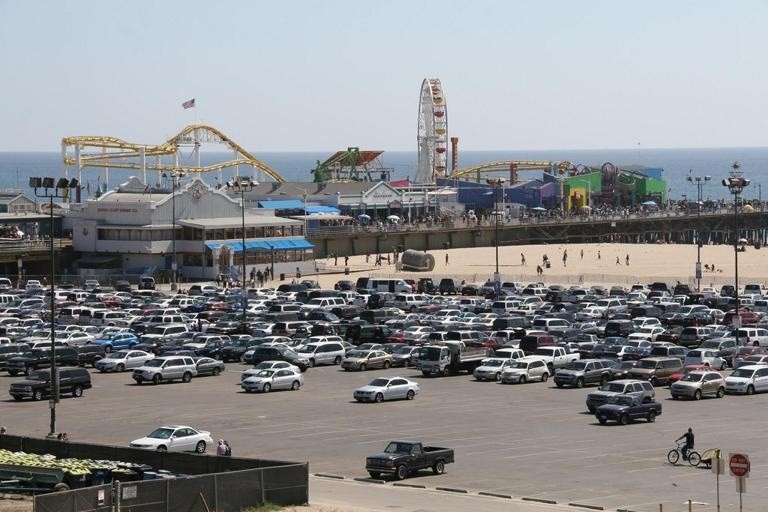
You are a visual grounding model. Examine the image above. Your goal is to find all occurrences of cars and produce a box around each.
[417,280,553,354]
[473,357,514,382]
[352,376,420,403]
[0,274,245,385]
[127,425,213,455]
[241,360,301,382]
[552,283,767,401]
[246,276,417,371]
[241,367,303,394]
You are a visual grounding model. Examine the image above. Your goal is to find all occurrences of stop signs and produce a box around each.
[727,451,750,479]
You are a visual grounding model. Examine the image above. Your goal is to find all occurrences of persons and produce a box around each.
[58,433,64,441]
[64,433,68,442]
[675,428,694,458]
[506,194,767,277]
[1,426,6,435]
[216,273,241,288]
[217,439,229,456]
[224,441,231,456]
[250,266,272,285]
[319,205,480,267]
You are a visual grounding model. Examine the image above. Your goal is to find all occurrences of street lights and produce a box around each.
[485,176,504,280]
[684,174,711,290]
[226,177,260,334]
[160,169,187,293]
[28,173,79,441]
[721,162,753,343]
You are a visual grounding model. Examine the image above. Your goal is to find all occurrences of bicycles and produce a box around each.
[667,440,700,467]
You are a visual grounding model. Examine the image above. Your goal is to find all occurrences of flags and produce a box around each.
[182,98,196,109]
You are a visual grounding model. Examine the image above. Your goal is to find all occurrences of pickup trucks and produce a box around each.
[594,394,662,426]
[364,440,454,480]
[522,346,580,378]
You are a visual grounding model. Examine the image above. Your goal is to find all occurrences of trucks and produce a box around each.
[417,341,488,377]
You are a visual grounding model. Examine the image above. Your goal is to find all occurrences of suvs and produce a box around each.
[494,349,524,361]
[8,366,91,401]
[586,379,656,415]
[501,358,550,385]
